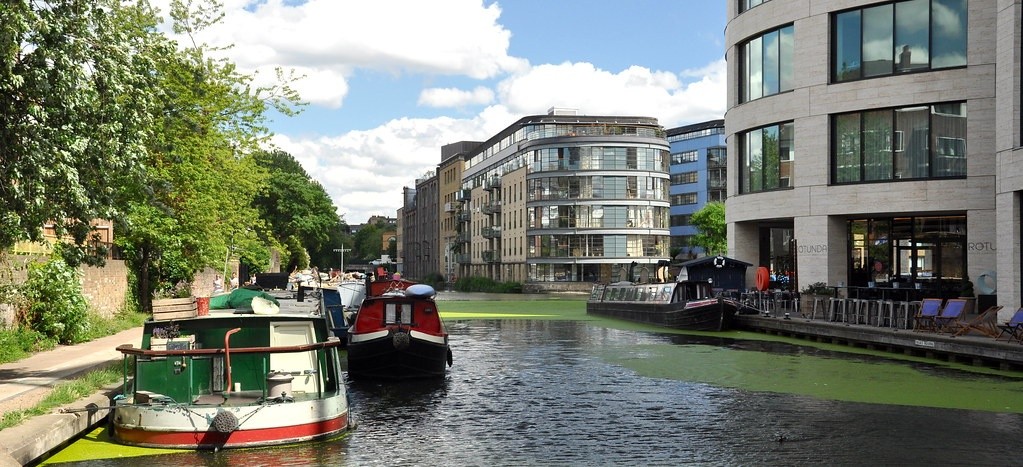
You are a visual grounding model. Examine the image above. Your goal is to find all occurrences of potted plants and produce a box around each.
[800,282,837,318]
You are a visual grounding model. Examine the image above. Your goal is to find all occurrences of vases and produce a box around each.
[151,296,198,321]
[151,332,196,350]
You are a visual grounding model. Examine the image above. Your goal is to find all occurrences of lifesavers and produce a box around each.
[713,256,726,269]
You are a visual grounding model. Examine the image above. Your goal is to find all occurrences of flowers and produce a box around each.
[151,277,191,298]
[153,321,179,338]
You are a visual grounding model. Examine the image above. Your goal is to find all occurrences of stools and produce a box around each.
[810,297,921,330]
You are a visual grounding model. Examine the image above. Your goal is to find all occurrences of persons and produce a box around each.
[770,270,790,282]
[228,272,239,289]
[250,274,257,283]
[213,275,224,292]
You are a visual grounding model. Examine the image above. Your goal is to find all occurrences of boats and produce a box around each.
[346,267,453,384]
[259,265,367,343]
[584,280,738,332]
[106,284,360,452]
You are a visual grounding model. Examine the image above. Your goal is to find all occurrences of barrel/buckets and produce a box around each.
[196,297,210,315]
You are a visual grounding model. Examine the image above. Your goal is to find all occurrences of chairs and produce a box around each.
[912,297,1023,344]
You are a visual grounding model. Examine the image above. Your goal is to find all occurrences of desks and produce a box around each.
[879,287,916,327]
[828,285,858,322]
[855,286,879,325]
[741,291,793,311]
